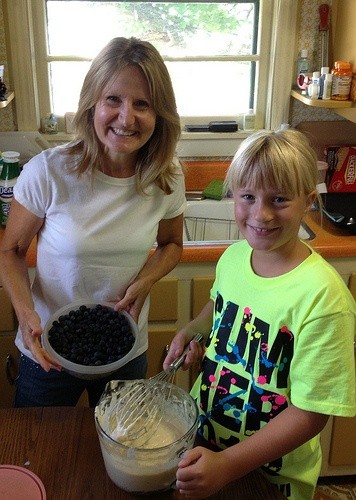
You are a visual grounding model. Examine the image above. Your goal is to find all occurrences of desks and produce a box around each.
[0,407,289,500]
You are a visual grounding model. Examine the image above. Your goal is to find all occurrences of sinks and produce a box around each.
[154,191,314,245]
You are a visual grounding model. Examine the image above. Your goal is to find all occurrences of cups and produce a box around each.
[94,378,199,495]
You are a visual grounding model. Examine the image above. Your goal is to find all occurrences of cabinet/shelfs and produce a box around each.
[0,260,356,478]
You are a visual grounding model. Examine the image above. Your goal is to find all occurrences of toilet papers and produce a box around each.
[281,124,289,129]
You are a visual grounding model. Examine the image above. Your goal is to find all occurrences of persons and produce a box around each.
[163,129,356,500]
[0,37,186,408]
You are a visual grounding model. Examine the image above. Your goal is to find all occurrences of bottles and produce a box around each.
[243,109,256,131]
[296,48,352,101]
[0,151,22,229]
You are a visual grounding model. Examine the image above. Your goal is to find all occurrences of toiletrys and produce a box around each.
[331,64,352,100]
[293,49,311,89]
[313,67,332,99]
[331,61,344,74]
[301,79,318,99]
[45,114,57,134]
[243,109,256,131]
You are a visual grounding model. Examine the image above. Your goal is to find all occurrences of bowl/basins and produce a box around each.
[42,299,138,379]
[317,161,328,184]
